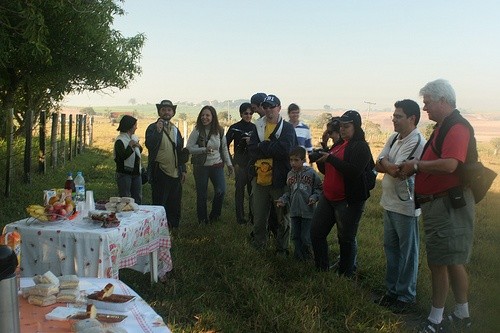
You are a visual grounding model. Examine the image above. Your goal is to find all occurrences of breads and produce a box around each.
[105,197,140,212]
[22,270,80,306]
[70,283,134,323]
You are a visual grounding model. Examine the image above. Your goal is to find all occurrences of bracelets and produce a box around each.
[379,157,384,163]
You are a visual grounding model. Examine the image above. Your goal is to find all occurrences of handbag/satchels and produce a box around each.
[472,167,497,203]
[142,168,148,184]
[181,148,189,163]
[255,158,273,186]
[366,157,377,190]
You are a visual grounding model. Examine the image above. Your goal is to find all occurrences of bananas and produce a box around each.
[26,205,48,221]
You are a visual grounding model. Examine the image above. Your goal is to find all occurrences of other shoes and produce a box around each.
[419,320,446,333]
[389,300,417,314]
[237,219,247,225]
[375,293,398,308]
[448,314,472,328]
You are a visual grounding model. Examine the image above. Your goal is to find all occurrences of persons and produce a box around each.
[186,105,233,224]
[225,103,260,224]
[115,115,143,205]
[145,100,187,239]
[375,99,427,314]
[251,92,268,142]
[322,116,341,151]
[394,79,476,333]
[247,94,295,241]
[310,110,370,278]
[274,145,322,273]
[287,102,314,167]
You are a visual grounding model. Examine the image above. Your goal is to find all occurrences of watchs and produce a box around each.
[414,160,420,173]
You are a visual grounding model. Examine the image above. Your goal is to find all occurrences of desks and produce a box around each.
[19,277,172,333]
[2,203,173,284]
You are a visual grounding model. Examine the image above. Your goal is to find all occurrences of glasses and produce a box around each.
[244,112,253,115]
[263,105,275,109]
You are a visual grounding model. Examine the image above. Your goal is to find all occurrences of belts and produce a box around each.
[419,190,449,204]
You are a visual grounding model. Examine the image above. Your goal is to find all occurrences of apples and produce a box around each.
[46,196,75,221]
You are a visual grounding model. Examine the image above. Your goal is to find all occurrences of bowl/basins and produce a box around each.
[83,216,120,228]
[66,310,128,329]
[87,290,137,312]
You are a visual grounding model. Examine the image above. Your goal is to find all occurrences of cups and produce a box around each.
[78,191,95,216]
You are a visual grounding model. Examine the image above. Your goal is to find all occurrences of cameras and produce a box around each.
[163,121,168,125]
[309,147,329,163]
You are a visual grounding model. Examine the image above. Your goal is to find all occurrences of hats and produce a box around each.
[156,100,177,118]
[335,110,361,124]
[261,95,280,107]
[327,117,341,132]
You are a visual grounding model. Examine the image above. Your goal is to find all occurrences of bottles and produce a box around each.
[406,176,414,200]
[64,172,76,214]
[74,172,85,212]
